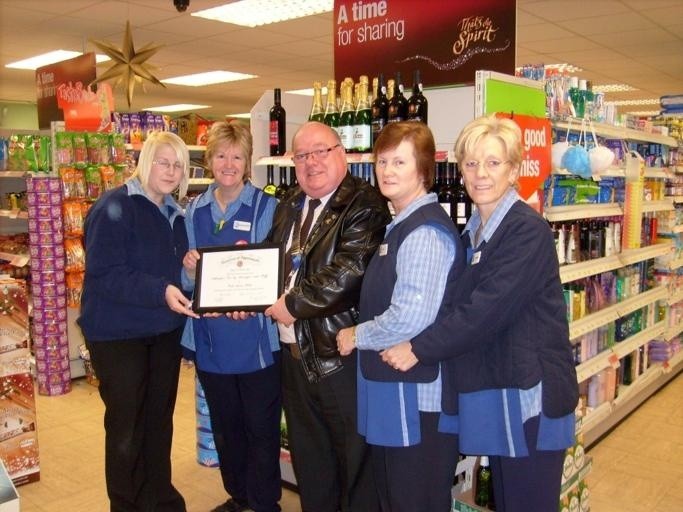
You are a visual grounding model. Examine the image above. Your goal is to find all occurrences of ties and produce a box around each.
[284,200,321,278]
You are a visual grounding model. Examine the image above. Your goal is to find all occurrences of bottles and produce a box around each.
[287,167,297,188]
[430,162,441,198]
[371,77,379,102]
[262,165,276,197]
[441,164,455,220]
[455,159,471,232]
[386,80,395,99]
[268,88,287,157]
[407,70,427,126]
[371,73,389,143]
[352,83,361,110]
[388,71,408,124]
[274,166,290,200]
[324,80,340,138]
[355,76,372,152]
[362,163,371,185]
[338,83,354,154]
[309,82,325,124]
[351,163,359,178]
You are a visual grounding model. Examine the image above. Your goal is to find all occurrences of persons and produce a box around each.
[179,121,282,511]
[379,113,581,512]
[336,120,462,512]
[226,121,392,512]
[76,133,189,512]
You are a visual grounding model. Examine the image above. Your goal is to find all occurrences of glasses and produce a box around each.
[466,159,511,167]
[296,145,339,159]
[153,158,181,169]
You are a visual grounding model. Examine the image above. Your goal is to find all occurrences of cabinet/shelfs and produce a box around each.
[248,70,592,512]
[540,118,683,451]
[123,143,215,214]
[0,121,86,382]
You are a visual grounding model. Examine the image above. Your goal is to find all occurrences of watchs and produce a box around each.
[351,326,357,349]
